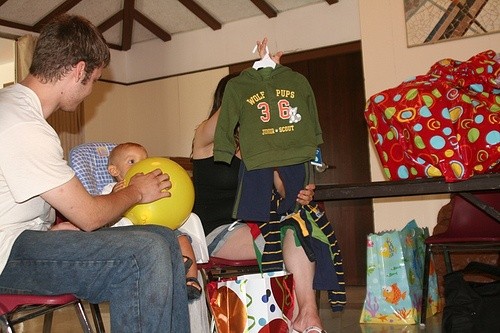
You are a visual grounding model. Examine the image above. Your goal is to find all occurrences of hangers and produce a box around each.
[252,43,276,70]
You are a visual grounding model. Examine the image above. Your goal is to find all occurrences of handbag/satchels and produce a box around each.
[359,222,439,323]
[441,262,500,333]
[362,47,500,182]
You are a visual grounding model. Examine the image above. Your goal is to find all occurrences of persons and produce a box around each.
[192,37,328,333]
[102,144,202,299]
[0,14,190,333]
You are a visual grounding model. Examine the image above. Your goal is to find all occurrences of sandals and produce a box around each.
[186,277,202,299]
[183,256,194,273]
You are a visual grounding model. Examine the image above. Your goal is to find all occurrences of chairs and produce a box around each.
[421,189,500,325]
[0,294,105,333]
[69,142,210,333]
[198,255,261,281]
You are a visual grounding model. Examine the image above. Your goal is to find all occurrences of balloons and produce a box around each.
[123,157,195,230]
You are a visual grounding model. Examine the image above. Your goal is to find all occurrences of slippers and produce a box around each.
[291,323,324,333]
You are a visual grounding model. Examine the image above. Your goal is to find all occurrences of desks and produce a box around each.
[312,172,500,202]
